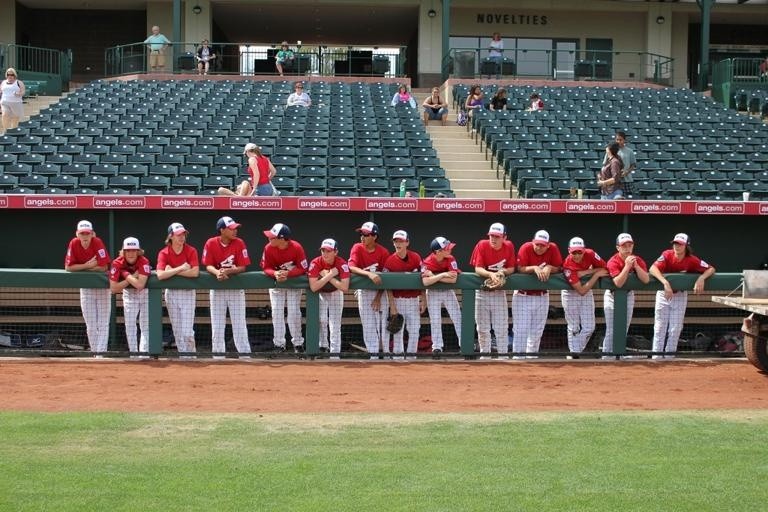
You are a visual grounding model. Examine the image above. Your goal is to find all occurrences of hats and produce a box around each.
[487,223,506,237]
[568,236,585,252]
[167,222,189,236]
[355,221,379,235]
[392,230,408,241]
[430,237,456,251]
[216,215,242,229]
[243,143,257,155]
[122,236,140,250]
[320,238,338,251]
[616,232,634,245]
[670,232,690,245]
[534,229,549,246]
[76,219,93,234]
[263,222,292,240]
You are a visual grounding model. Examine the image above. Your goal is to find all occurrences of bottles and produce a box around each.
[419,182,425,197]
[399,178,406,198]
[570,186,576,200]
[578,189,583,199]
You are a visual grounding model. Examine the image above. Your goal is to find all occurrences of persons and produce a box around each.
[490,87,507,111]
[469,223,516,361]
[287,83,311,107]
[109,237,151,358]
[259,223,311,359]
[143,25,171,71]
[759,57,768,82]
[420,236,476,358]
[274,42,295,76]
[65,220,111,359]
[382,230,427,360]
[485,33,504,80]
[194,39,216,75]
[348,222,387,359]
[525,92,544,111]
[307,238,349,359]
[510,230,564,361]
[603,132,638,200]
[0,67,26,133]
[154,223,199,359]
[597,142,625,202]
[391,84,416,108]
[465,85,484,117]
[602,232,650,358]
[218,180,252,197]
[202,217,264,361]
[650,233,714,358]
[562,237,608,359]
[243,142,277,199]
[423,87,448,126]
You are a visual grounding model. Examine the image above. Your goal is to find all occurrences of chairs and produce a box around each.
[454,86,767,199]
[0,78,453,197]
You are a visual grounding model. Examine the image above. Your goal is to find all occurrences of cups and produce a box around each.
[570,187,576,199]
[742,192,749,203]
[577,188,583,199]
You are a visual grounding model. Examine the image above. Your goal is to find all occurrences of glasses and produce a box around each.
[297,86,303,89]
[359,233,369,237]
[6,74,15,76]
